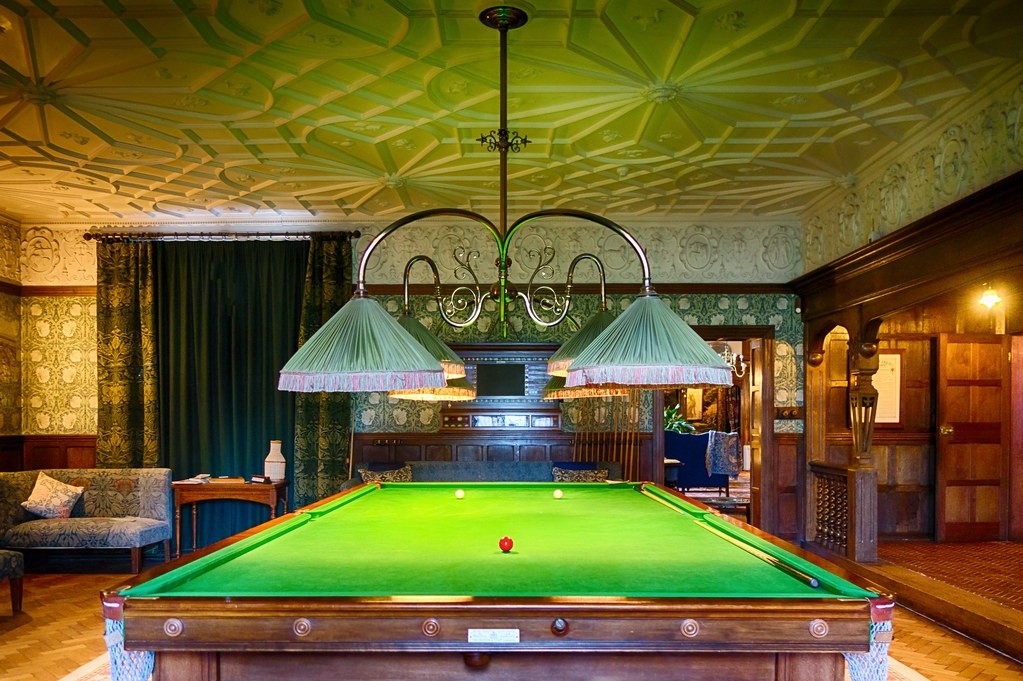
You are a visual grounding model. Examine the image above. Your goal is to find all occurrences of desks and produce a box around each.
[172,475,288,559]
[102,481,896,679]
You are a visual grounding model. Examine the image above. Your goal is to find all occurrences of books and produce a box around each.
[251,475,272,484]
[209,477,245,483]
[172,473,211,484]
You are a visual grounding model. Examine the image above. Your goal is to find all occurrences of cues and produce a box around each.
[348,397,357,481]
[632,485,685,516]
[692,519,819,589]
[572,390,641,482]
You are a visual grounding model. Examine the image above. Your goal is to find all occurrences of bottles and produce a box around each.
[264,440,286,482]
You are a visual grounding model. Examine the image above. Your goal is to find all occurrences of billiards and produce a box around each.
[553,489,562,498]
[454,489,465,499]
[498,537,513,551]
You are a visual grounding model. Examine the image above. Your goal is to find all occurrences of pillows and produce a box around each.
[20,470,84,518]
[551,467,609,483]
[357,464,413,483]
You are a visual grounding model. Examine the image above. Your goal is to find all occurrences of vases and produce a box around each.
[264,439,286,480]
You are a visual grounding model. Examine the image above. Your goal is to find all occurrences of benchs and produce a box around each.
[664,430,744,498]
[0,550,24,612]
[0,468,172,574]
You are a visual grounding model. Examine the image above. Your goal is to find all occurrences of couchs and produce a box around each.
[338,459,623,492]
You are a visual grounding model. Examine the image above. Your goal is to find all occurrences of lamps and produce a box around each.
[716,341,747,379]
[979,285,1001,307]
[277,5,736,402]
